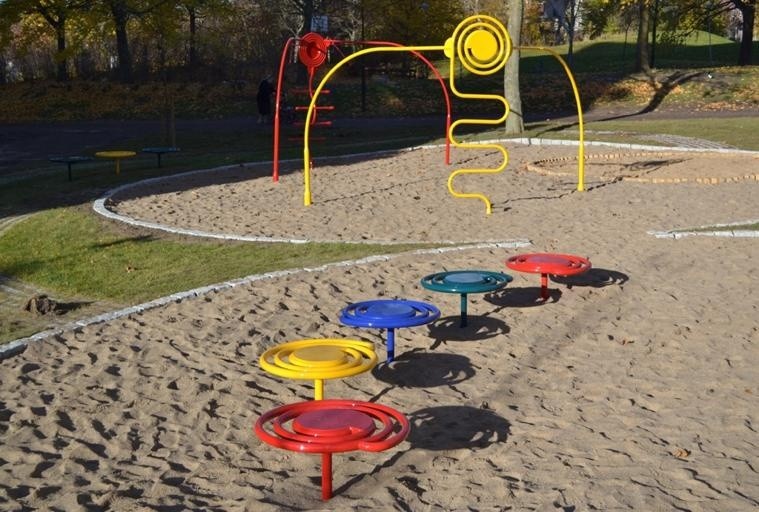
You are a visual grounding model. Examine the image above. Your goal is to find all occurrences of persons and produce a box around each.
[254,74,276,125]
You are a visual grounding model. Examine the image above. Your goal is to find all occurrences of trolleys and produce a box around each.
[274,90,299,124]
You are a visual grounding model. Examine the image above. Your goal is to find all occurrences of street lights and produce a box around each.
[703,1,713,67]
